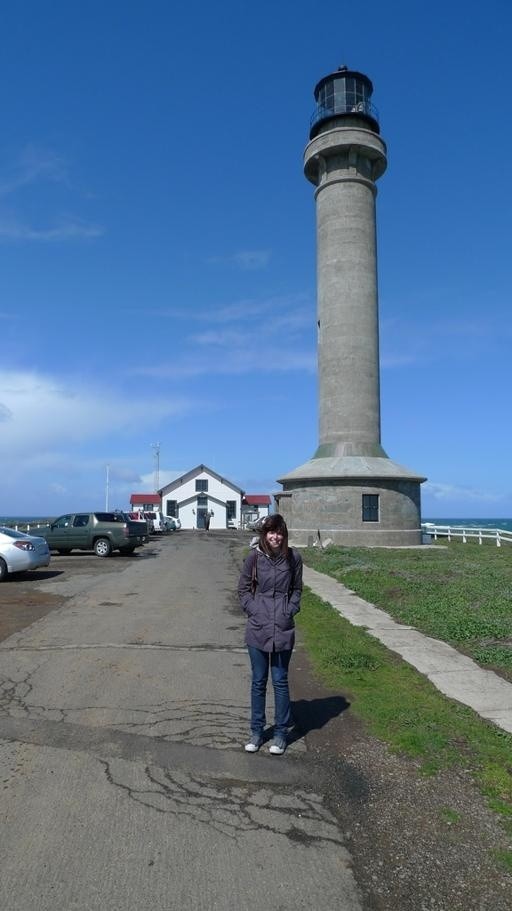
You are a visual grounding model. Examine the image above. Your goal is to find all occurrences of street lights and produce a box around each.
[151,440,160,493]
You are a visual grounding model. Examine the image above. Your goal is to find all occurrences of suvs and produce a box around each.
[25,511,148,562]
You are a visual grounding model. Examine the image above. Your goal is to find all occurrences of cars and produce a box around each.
[120,509,180,534]
[245,515,272,531]
[0,526,50,576]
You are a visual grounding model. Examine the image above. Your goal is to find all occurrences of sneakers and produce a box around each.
[244,735,264,753]
[269,734,290,755]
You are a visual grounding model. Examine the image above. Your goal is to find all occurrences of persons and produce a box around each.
[205,512,210,532]
[237,514,304,757]
